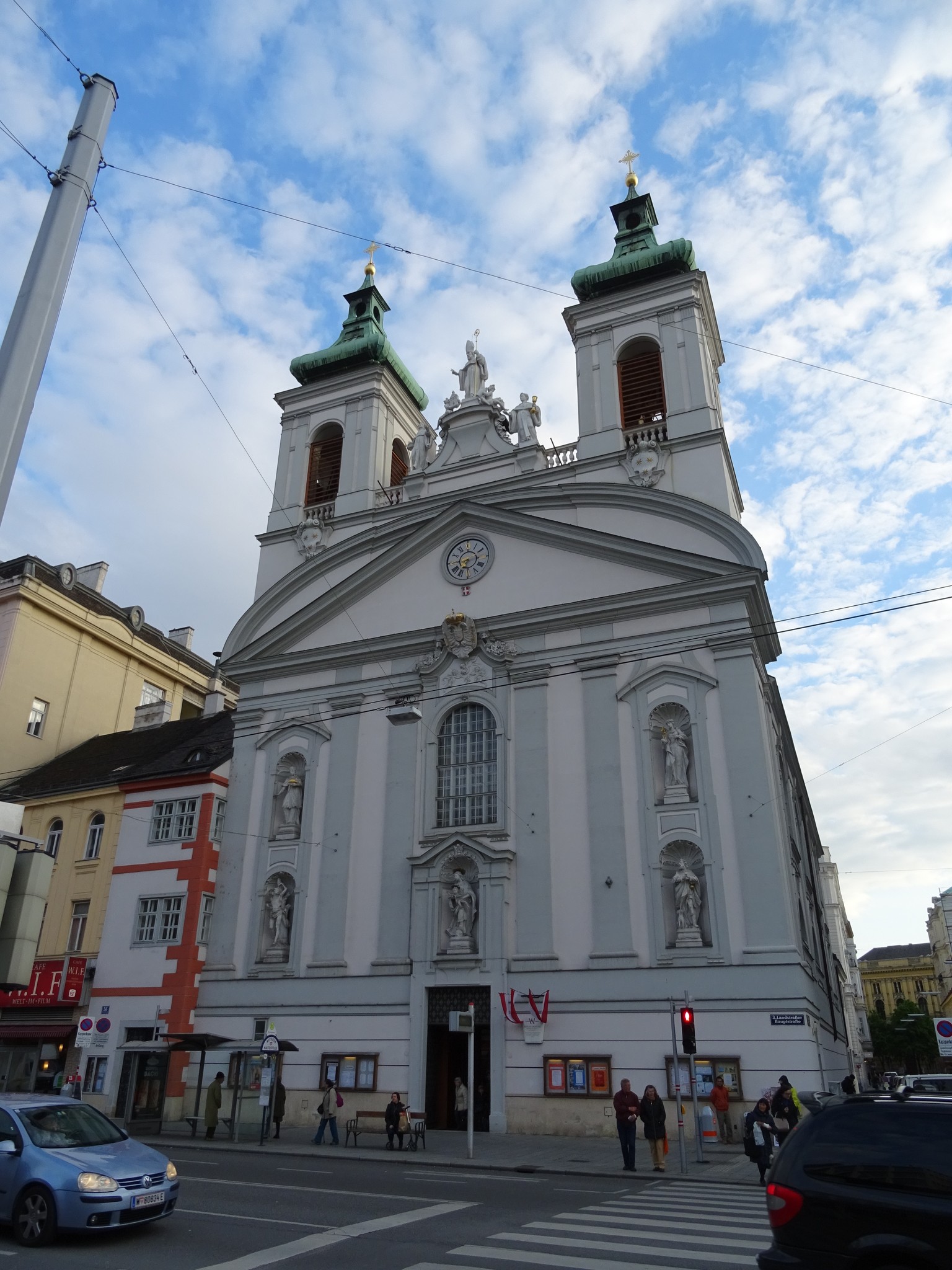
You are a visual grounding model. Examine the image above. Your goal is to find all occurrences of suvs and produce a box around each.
[883,1072,952,1094]
[756,1091,952,1270]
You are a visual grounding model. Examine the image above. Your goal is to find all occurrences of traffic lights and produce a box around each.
[680,1007,697,1054]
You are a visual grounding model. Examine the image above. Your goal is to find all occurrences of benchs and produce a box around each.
[344,1108,427,1154]
[184,1116,230,1129]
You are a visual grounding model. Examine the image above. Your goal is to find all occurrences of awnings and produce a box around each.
[0,1025,76,1037]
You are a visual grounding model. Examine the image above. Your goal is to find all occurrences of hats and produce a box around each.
[215,1071,226,1079]
[778,1078,784,1084]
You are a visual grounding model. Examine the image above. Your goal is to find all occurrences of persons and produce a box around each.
[502,392,541,443]
[454,1077,468,1131]
[407,421,431,470]
[770,1075,802,1148]
[445,871,477,937]
[450,340,489,399]
[266,882,288,947]
[385,1092,405,1149]
[672,859,702,929]
[660,719,689,786]
[746,1098,778,1184]
[841,1074,856,1095]
[204,1071,226,1141]
[433,398,461,431]
[474,1085,488,1132]
[613,1078,667,1172]
[710,1076,736,1145]
[275,766,303,824]
[262,1075,286,1139]
[481,384,509,411]
[311,1079,339,1145]
[867,1069,897,1090]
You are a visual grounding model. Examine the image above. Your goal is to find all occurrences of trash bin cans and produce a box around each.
[60,1084,74,1098]
[701,1106,719,1143]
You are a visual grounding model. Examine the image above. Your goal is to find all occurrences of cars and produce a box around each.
[0,1092,179,1248]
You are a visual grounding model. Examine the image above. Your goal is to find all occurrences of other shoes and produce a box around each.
[205,1137,215,1140]
[726,1139,735,1144]
[399,1147,403,1149]
[760,1178,767,1184]
[273,1135,279,1138]
[390,1144,394,1148]
[658,1168,664,1172]
[330,1142,339,1145]
[653,1167,659,1170]
[312,1140,321,1145]
[629,1167,636,1171]
[722,1140,726,1144]
[623,1166,629,1170]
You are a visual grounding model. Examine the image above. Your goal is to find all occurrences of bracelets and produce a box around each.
[634,1114,636,1118]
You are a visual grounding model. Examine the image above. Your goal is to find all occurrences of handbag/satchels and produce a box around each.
[663,1122,668,1154]
[773,1112,789,1132]
[398,1111,411,1131]
[317,1102,324,1114]
[753,1121,765,1145]
[743,1137,754,1156]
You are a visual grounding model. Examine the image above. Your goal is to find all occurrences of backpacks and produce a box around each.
[328,1088,344,1107]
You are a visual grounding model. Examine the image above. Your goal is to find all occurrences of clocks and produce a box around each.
[442,534,496,586]
